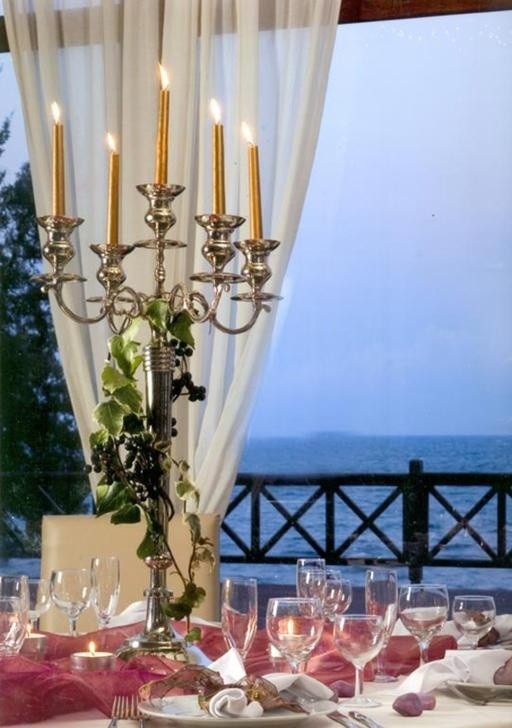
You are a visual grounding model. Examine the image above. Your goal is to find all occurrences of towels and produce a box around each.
[393,643,512,699]
[194,645,340,721]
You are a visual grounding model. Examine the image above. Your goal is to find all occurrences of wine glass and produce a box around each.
[333,614,381,709]
[222,576,257,664]
[263,560,398,683]
[452,594,495,650]
[1,557,122,658]
[398,584,450,668]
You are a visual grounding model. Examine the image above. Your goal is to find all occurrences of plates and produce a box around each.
[138,696,338,727]
[450,679,511,703]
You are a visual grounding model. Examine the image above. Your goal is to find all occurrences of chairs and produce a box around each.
[34,508,224,632]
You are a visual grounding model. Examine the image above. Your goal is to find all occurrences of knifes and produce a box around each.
[351,711,383,727]
[330,712,359,727]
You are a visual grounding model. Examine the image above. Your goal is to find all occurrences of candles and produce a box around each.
[106,132,121,244]
[240,123,264,240]
[209,97,227,213]
[156,63,171,185]
[52,103,65,216]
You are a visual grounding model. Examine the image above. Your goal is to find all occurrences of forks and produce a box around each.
[108,696,130,728]
[132,694,153,727]
